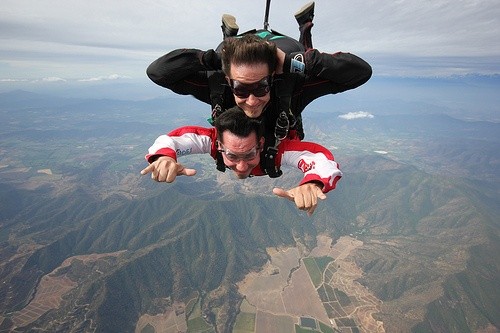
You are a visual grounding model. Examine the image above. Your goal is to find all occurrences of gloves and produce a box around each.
[204,49,220,70]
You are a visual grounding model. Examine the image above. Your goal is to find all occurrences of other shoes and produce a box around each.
[222,14,239,36]
[295,1,315,26]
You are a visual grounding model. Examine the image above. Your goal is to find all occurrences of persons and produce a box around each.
[140,106,344,216]
[146,1,372,141]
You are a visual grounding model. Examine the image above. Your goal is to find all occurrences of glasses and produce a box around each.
[222,144,258,161]
[230,76,272,99]
